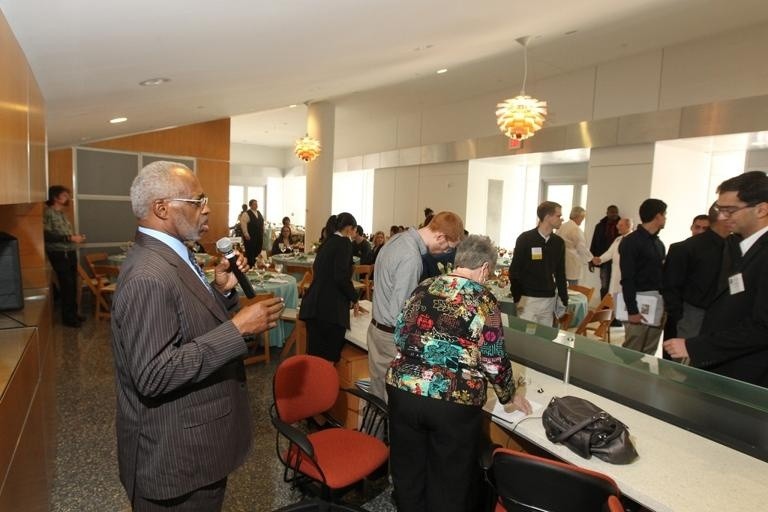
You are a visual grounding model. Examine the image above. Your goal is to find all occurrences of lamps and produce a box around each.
[294,132,323,166]
[494,33,547,142]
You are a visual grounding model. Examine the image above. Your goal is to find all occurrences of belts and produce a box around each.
[372,319,394,333]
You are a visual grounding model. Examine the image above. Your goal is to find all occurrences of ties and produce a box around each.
[187,248,214,296]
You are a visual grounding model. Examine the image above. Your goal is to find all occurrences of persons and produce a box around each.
[44,186,86,331]
[272,217,293,254]
[389,235,534,512]
[296,212,368,430]
[237,204,248,220]
[281,216,292,228]
[320,208,468,298]
[239,198,264,266]
[663,172,767,388]
[508,198,733,362]
[368,212,467,410]
[109,160,289,511]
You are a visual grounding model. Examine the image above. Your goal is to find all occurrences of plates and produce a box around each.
[115,255,128,259]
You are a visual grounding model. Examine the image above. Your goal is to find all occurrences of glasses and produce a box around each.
[175,196,209,209]
[713,201,748,214]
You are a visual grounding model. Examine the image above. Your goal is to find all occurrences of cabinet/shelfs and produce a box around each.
[1,286,57,512]
[0,8,50,207]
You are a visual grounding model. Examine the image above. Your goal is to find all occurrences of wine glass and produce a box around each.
[253,258,284,289]
[280,245,299,259]
[488,268,511,299]
[498,248,512,262]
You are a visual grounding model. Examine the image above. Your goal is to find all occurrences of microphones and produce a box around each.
[216,236,256,299]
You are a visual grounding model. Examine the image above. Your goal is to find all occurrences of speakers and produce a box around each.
[0,232,24,312]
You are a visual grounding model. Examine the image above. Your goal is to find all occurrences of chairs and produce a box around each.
[550,284,618,345]
[226,240,227,251]
[76,242,375,367]
[487,448,625,512]
[266,354,391,512]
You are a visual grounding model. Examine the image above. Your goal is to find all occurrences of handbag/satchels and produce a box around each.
[542,396,637,464]
